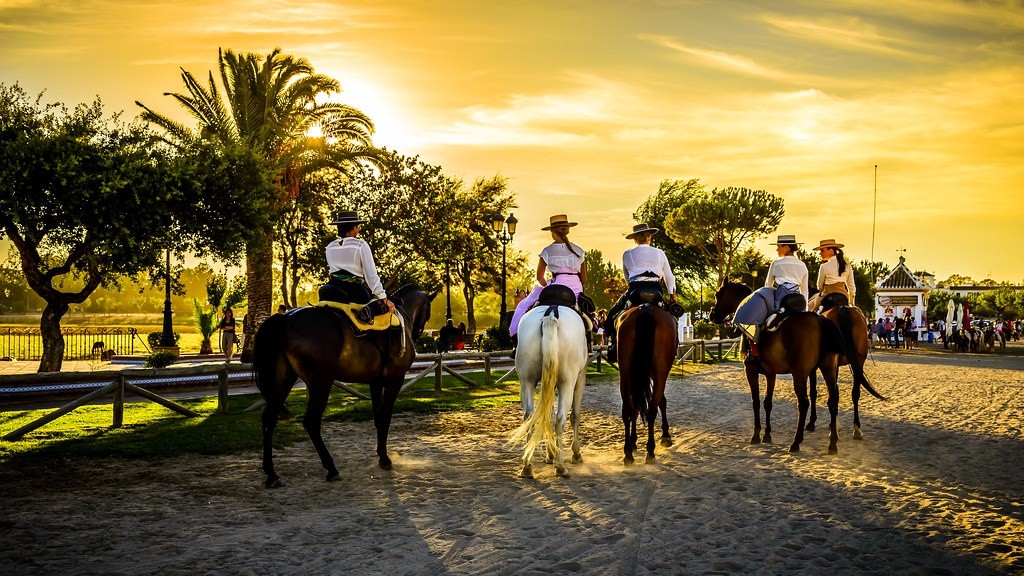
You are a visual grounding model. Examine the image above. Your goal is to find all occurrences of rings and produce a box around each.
[392,308,393,309]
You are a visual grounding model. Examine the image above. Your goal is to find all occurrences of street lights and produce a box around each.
[492,212,520,350]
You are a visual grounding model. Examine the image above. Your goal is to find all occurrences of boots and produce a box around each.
[510,334,519,358]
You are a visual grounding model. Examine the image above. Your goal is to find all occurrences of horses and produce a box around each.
[710,275,839,457]
[946,327,1006,353]
[807,301,888,441]
[252,256,439,489]
[612,298,681,463]
[509,303,590,479]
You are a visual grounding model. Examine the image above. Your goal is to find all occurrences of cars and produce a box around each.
[969,319,990,328]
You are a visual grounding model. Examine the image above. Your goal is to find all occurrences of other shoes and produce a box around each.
[742,353,761,363]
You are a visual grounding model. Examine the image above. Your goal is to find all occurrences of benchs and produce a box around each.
[432,334,483,352]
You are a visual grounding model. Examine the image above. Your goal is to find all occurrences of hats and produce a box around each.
[812,240,845,251]
[328,211,368,225]
[626,224,660,239]
[769,235,804,245]
[540,215,579,231]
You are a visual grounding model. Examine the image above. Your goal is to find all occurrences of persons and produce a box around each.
[731,234,808,364]
[220,309,235,363]
[601,223,677,362]
[808,239,856,312]
[979,317,1024,349]
[434,319,466,353]
[276,305,287,315]
[867,308,946,350]
[508,215,587,360]
[326,211,396,325]
[591,309,608,346]
